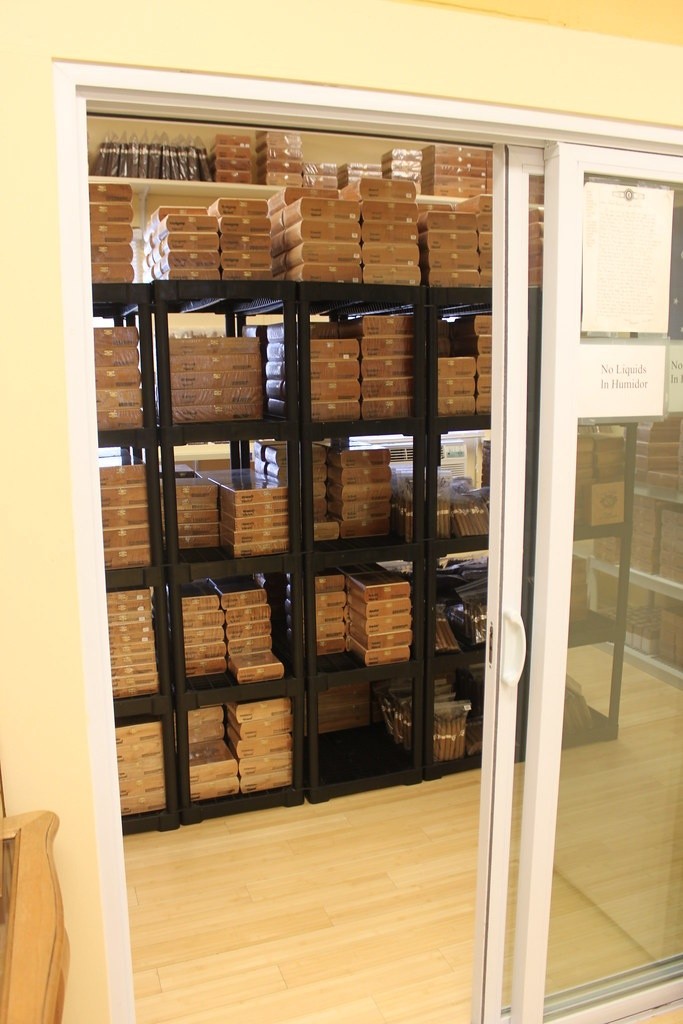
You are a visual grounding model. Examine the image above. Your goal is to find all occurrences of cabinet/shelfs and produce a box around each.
[86,128,683,836]
